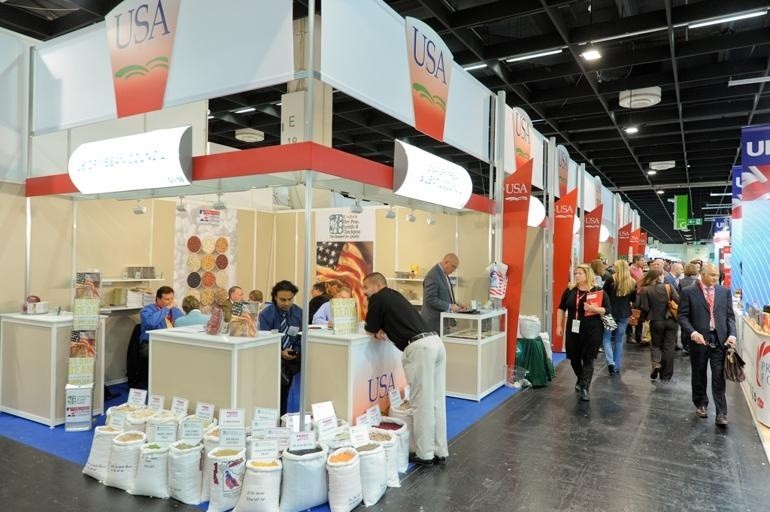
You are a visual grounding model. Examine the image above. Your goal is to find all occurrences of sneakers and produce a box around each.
[651,365,660,379]
[608,365,620,375]
[575,382,590,401]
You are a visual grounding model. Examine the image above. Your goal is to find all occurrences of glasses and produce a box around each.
[705,340,717,349]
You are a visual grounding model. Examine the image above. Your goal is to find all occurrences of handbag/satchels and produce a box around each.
[601,314,618,331]
[628,308,641,326]
[668,300,679,321]
[724,347,746,383]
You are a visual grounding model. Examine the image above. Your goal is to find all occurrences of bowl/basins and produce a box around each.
[182,235,230,314]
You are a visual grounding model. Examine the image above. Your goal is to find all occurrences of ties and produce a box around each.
[280,312,291,351]
[706,289,715,328]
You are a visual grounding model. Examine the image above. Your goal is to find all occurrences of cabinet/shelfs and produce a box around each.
[98,277,165,316]
[384,276,458,308]
[438,307,509,403]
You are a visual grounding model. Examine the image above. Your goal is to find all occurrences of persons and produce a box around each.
[248,289,271,321]
[257,280,302,427]
[309,283,329,324]
[174,295,212,327]
[361,272,450,467]
[421,254,468,334]
[555,254,747,428]
[312,286,352,327]
[222,285,246,321]
[138,286,184,359]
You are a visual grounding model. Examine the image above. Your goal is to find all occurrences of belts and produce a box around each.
[409,332,438,343]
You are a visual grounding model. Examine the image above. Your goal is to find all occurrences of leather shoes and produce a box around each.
[409,452,447,467]
[716,415,729,425]
[696,405,708,418]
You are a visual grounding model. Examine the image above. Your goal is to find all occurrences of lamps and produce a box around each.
[649,160,675,171]
[622,89,640,136]
[234,127,265,142]
[646,167,667,196]
[391,137,475,211]
[578,0,603,62]
[67,125,197,197]
[526,195,610,243]
[618,85,662,109]
